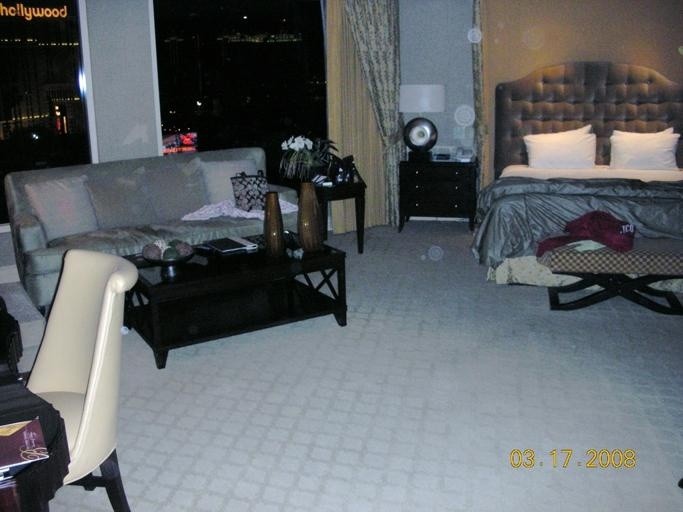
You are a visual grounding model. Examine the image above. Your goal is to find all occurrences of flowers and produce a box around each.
[281,134,340,180]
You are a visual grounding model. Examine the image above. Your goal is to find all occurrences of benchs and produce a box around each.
[546,237,683,311]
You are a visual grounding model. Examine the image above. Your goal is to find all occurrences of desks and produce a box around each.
[266,170,367,255]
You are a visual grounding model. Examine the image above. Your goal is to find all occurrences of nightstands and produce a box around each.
[398,159,479,234]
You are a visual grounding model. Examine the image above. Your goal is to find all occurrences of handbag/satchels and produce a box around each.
[230,175,269,211]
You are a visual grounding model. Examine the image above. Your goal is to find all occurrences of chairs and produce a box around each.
[24,247,140,511]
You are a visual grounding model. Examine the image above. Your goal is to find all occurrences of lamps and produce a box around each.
[398,83,446,163]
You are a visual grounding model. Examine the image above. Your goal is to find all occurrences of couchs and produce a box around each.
[4,146,299,318]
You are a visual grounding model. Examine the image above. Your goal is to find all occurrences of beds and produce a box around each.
[493,59,682,294]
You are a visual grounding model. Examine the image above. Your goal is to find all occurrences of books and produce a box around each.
[0,417,49,490]
[203,234,258,254]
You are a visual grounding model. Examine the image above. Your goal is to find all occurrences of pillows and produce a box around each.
[23,158,258,243]
[522,123,681,173]
